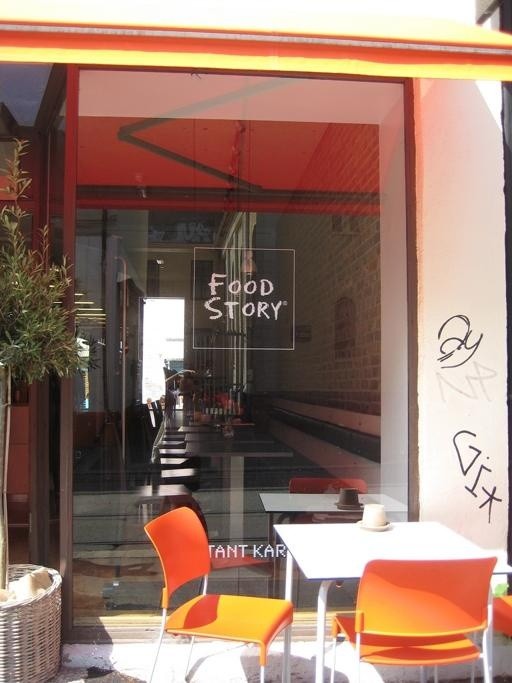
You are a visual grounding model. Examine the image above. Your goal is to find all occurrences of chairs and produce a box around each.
[277,477,368,609]
[158,492,280,598]
[110,426,208,610]
[143,505,294,683]
[330,557,496,682]
[420,594,512,682]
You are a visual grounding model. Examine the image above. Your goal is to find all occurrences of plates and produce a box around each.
[334,503,364,509]
[357,520,391,532]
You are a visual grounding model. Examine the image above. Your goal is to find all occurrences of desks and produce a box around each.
[257,492,408,599]
[271,522,512,683]
[178,410,294,580]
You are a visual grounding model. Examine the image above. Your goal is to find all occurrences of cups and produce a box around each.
[363,503,386,526]
[338,487,358,505]
